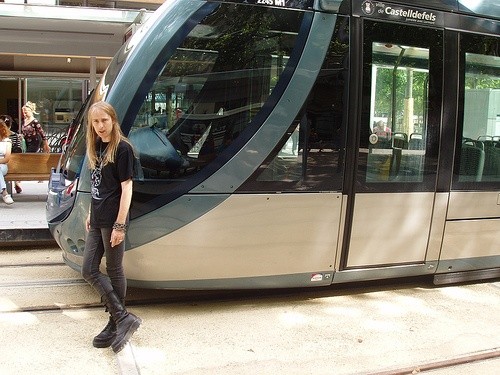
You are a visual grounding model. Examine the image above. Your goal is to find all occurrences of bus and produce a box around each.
[44,0,500,291]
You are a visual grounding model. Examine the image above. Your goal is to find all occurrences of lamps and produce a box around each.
[24,0,27,3]
[66,58,72,63]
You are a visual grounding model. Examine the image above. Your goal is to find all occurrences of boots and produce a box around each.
[93,297,126,348]
[100,291,141,354]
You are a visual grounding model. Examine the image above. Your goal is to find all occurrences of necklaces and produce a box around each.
[99,141,110,162]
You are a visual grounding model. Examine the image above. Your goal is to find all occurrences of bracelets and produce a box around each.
[113,223,126,231]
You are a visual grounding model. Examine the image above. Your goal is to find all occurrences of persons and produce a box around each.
[81,102,142,354]
[0,102,48,204]
[374,120,392,137]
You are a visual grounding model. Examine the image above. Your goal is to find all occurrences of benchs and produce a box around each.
[5,153,61,196]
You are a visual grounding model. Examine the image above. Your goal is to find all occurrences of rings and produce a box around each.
[120,240,123,242]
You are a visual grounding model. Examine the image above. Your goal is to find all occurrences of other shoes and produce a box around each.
[15,184,22,194]
[3,195,13,204]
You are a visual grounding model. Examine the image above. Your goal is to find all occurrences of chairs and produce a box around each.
[197,111,236,160]
[367,131,500,182]
